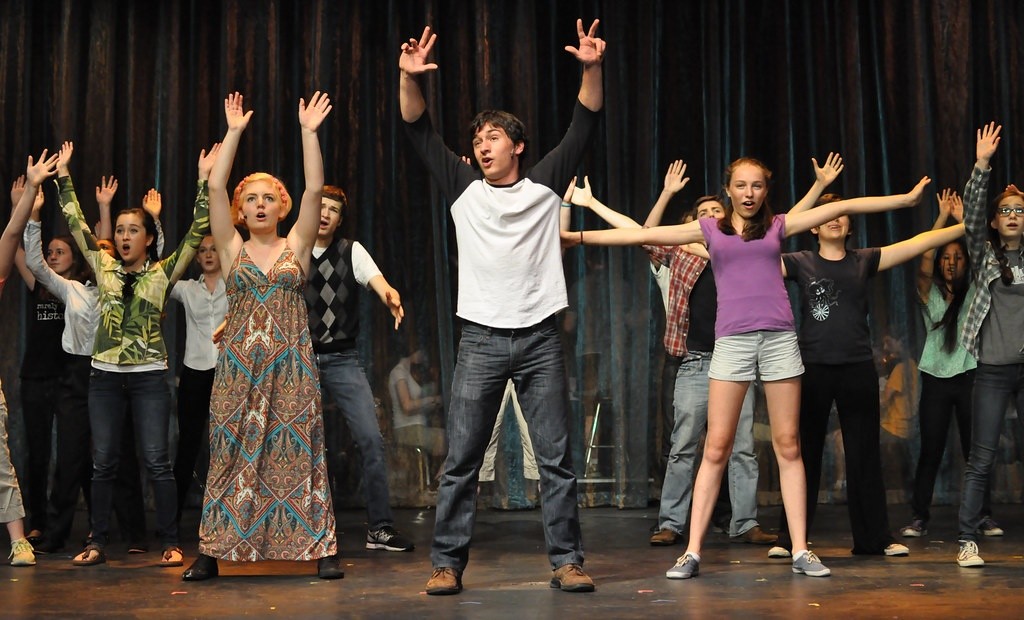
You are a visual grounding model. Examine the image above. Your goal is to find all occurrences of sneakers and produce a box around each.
[650,529,683,545]
[365,528,414,551]
[884,543,910,556]
[730,525,778,544]
[792,550,830,577]
[8,537,37,566]
[549,562,595,591]
[425,567,463,594]
[956,539,984,567]
[976,518,1004,536]
[666,553,701,578]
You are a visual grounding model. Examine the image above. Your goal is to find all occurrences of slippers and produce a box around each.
[159,546,183,567]
[71,544,107,566]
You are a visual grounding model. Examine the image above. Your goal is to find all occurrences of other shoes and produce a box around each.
[318,554,344,579]
[127,543,148,554]
[33,537,65,555]
[930,535,944,543]
[180,553,220,580]
[768,537,792,558]
[68,537,92,559]
[900,518,923,537]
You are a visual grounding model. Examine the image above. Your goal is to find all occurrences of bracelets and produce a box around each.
[580,231,582,244]
[562,204,571,207]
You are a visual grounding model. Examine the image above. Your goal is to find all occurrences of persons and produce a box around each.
[957,121,1024,566]
[182,92,346,581]
[560,152,966,580]
[900,188,1005,536]
[211,185,415,554]
[0,140,229,566]
[399,19,606,595]
[389,243,921,508]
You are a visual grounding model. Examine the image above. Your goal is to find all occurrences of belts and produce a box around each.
[474,314,556,339]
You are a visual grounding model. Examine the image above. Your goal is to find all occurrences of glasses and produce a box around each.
[997,207,1024,216]
[121,273,136,298]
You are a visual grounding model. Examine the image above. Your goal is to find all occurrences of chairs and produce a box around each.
[382,377,431,510]
